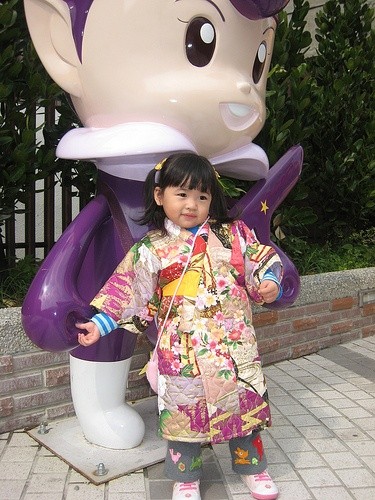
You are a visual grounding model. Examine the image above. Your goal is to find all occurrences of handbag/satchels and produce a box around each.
[146,352,157,396]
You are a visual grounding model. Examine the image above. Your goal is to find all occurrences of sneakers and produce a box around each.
[239,467,278,499]
[171,476,202,500]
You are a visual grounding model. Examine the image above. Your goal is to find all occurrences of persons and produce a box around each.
[74,152,286,499]
[22,0,303,449]
